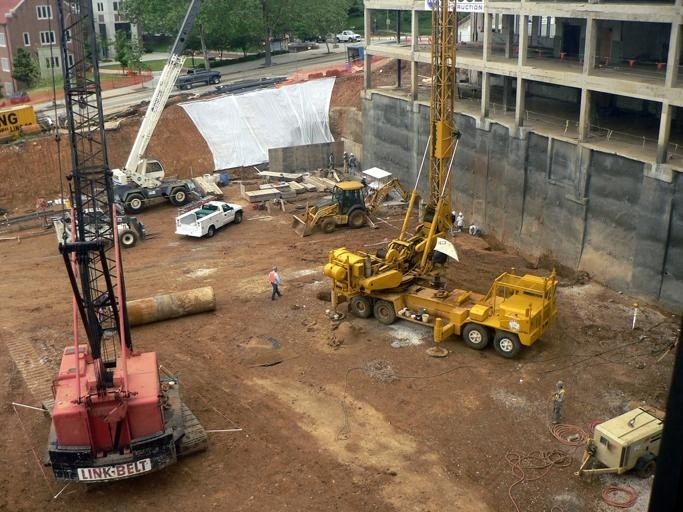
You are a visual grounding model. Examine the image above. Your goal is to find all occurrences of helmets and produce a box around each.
[555,380,566,388]
[452,210,473,230]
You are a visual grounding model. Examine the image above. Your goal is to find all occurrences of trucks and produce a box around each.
[297,30,328,43]
[0,107,53,142]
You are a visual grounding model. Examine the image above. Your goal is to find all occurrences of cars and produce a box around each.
[303,41,321,49]
[9,89,32,105]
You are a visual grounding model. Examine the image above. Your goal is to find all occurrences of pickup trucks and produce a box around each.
[173,195,244,238]
[170,66,225,89]
[333,29,361,43]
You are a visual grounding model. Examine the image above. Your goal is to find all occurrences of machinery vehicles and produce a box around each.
[51,210,159,249]
[101,0,207,216]
[38,0,211,485]
[320,0,564,357]
[288,176,419,239]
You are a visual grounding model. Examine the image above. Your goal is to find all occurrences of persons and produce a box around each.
[268,266,283,301]
[455,212,464,232]
[344,151,349,172]
[329,151,334,170]
[349,153,356,173]
[345,61,350,73]
[452,211,456,226]
[552,381,564,426]
[468,226,480,236]
